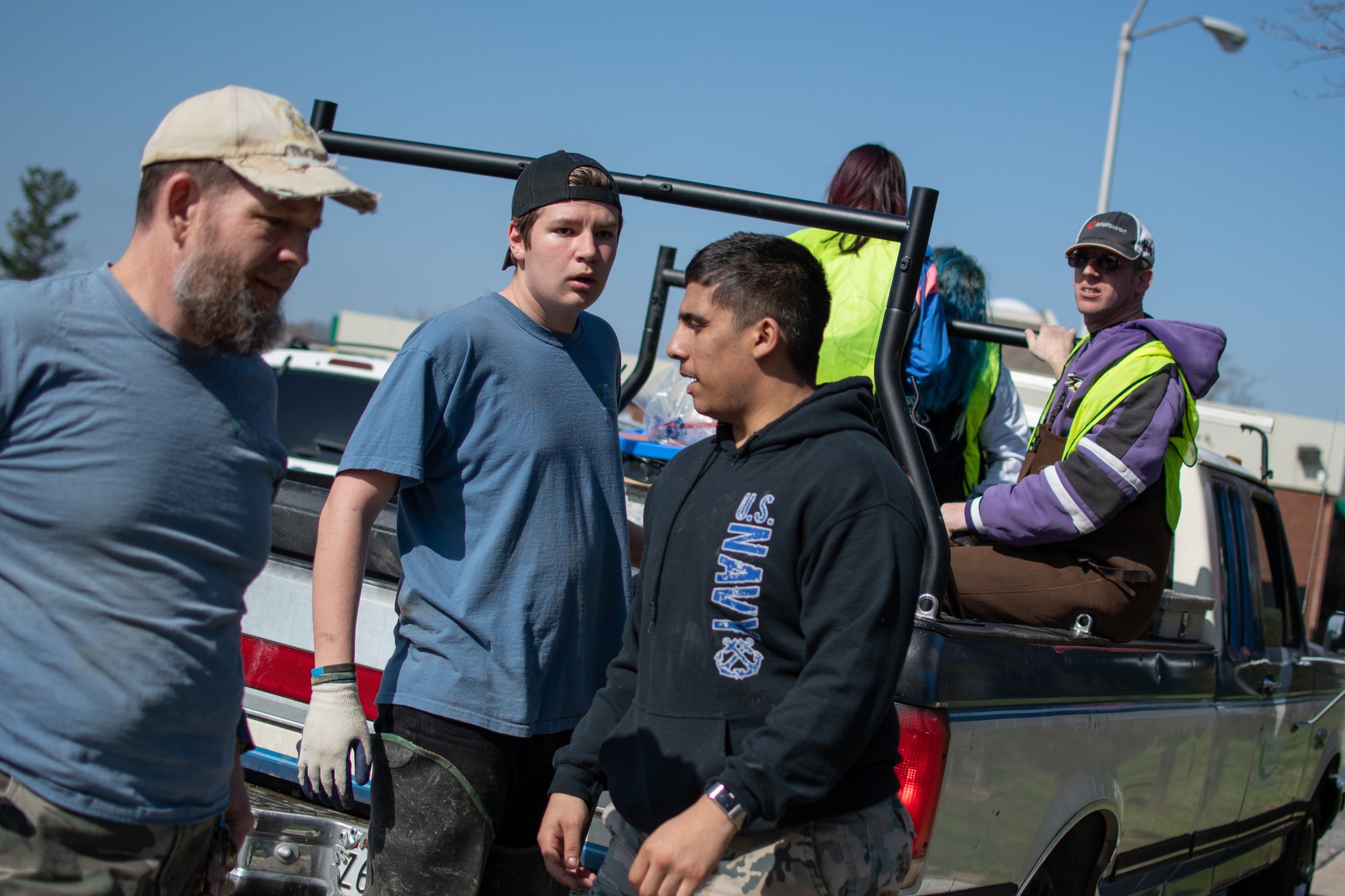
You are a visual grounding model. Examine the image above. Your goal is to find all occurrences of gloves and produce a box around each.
[296,663,374,810]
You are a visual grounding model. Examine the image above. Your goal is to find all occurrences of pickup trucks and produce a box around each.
[236,369,1345,895]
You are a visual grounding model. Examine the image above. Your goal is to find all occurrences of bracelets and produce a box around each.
[310,661,356,678]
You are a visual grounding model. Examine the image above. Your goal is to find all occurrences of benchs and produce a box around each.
[898,617,1216,707]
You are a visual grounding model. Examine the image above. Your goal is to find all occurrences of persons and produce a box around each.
[307,151,631,896]
[777,143,952,431]
[549,233,930,896]
[935,206,1227,641]
[1,84,376,896]
[915,249,1030,505]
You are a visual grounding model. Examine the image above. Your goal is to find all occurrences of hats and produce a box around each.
[501,150,622,271]
[1065,211,1155,268]
[141,84,378,214]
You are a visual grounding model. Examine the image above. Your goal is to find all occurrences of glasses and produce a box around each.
[1066,250,1136,273]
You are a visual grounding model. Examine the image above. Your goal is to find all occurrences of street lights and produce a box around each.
[1094,0,1247,217]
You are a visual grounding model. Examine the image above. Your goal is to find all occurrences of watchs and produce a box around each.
[707,780,746,832]
